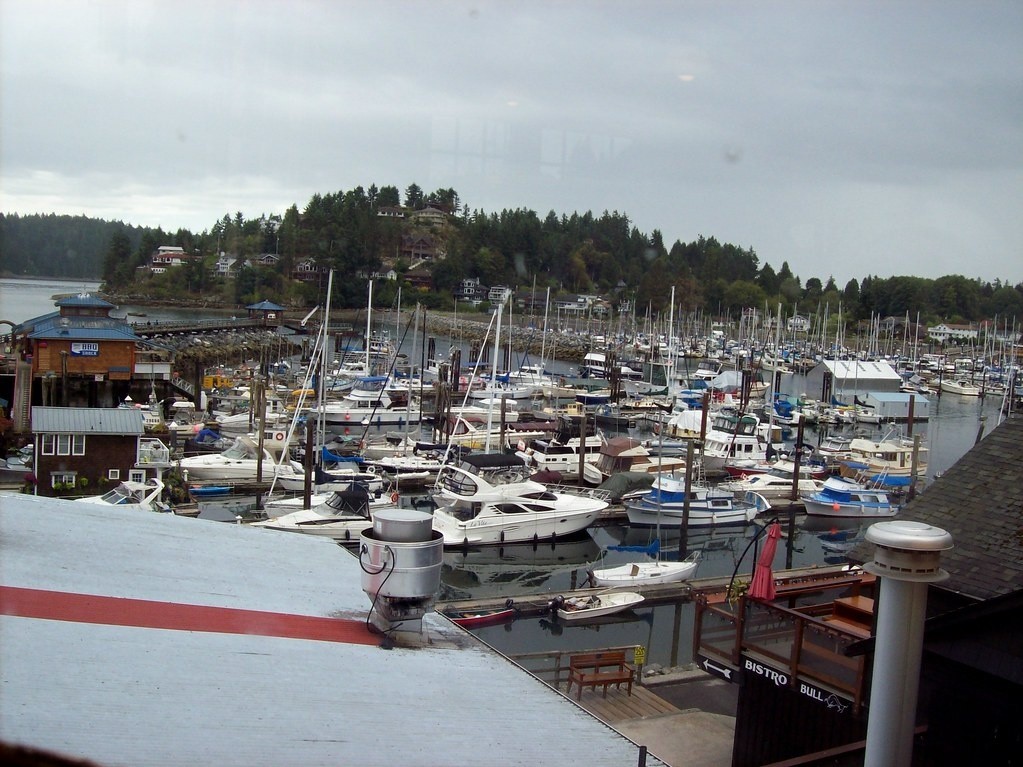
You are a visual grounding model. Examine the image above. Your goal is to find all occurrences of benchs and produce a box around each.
[566,650,636,702]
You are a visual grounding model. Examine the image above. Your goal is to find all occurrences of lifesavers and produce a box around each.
[276,433,284,441]
[391,493,399,503]
[368,466,376,474]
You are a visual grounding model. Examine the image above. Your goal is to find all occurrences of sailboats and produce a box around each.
[307,279,426,424]
[590,404,704,585]
[704,300,848,373]
[534,299,702,357]
[430,288,532,508]
[360,302,451,504]
[725,304,825,480]
[263,270,401,520]
[276,336,385,492]
[849,308,1023,397]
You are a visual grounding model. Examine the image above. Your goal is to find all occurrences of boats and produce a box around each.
[171,435,295,488]
[332,353,566,400]
[801,461,907,517]
[122,367,292,434]
[251,474,399,543]
[578,436,695,487]
[547,592,644,619]
[719,455,830,509]
[440,530,606,597]
[569,349,725,409]
[431,464,609,545]
[815,525,874,560]
[72,476,175,515]
[837,438,931,486]
[693,416,787,477]
[441,606,515,624]
[607,523,759,555]
[619,469,770,526]
[820,436,856,469]
